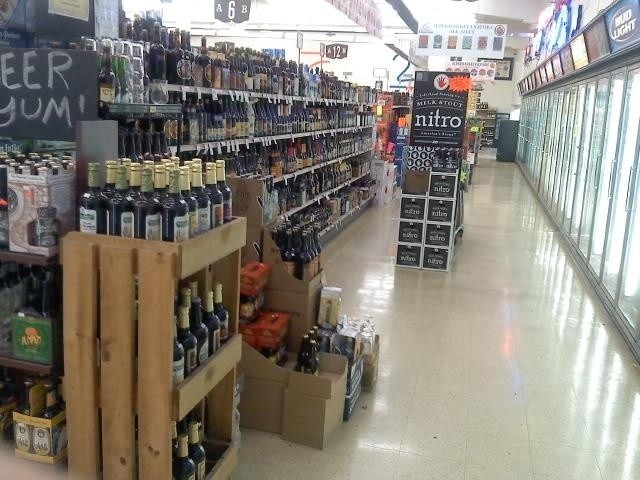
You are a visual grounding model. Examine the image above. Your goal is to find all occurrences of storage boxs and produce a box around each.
[395,167,460,272]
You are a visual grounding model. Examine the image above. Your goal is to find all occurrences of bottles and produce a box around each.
[173,277,230,385]
[0,370,66,418]
[0,265,64,320]
[239,290,376,377]
[433,146,459,169]
[0,11,380,279]
[171,416,207,480]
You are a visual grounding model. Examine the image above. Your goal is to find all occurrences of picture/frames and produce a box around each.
[478,57,514,80]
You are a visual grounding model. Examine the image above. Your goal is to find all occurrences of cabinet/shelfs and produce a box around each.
[0,1,410,480]
